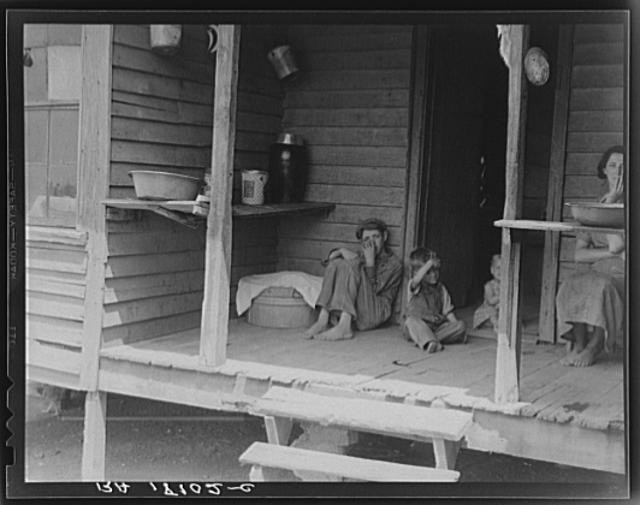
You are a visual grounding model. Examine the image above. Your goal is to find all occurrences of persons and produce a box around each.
[400,247,469,354]
[472,254,501,332]
[555,146,626,368]
[300,218,404,341]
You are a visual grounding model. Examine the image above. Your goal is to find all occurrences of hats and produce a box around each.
[355,218,387,239]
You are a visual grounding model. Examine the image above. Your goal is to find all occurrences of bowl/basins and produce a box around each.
[238,283,323,328]
[128,169,202,199]
[273,131,305,147]
[564,200,626,226]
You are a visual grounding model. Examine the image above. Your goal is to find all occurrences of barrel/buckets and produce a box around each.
[242,168,269,206]
[268,44,298,80]
